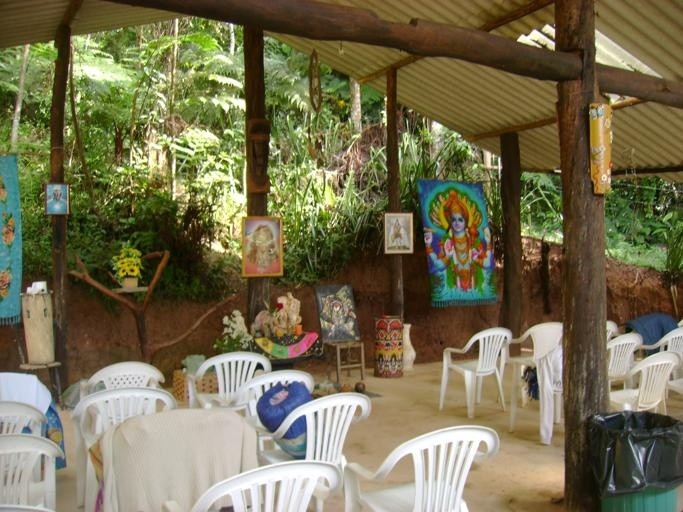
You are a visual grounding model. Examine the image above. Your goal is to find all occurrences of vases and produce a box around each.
[122,277,139,288]
[401,322,416,373]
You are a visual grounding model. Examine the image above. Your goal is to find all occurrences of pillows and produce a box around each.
[255,380,318,459]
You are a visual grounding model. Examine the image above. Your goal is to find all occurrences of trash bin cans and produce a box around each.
[587,410,683,512]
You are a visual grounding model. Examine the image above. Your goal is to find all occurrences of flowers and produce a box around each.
[111,240,144,279]
[213,309,253,355]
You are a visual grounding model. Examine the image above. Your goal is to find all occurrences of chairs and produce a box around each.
[69,386,178,512]
[534,320,618,446]
[233,369,315,451]
[160,458,342,512]
[0,433,65,507]
[639,327,683,380]
[606,331,643,393]
[438,327,513,419]
[186,350,272,417]
[0,401,49,482]
[257,392,373,512]
[10,321,65,412]
[343,424,500,512]
[80,360,166,435]
[313,281,366,382]
[608,351,680,415]
[495,321,564,434]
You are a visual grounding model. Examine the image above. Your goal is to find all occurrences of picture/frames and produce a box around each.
[384,212,415,255]
[44,183,71,215]
[241,216,284,278]
